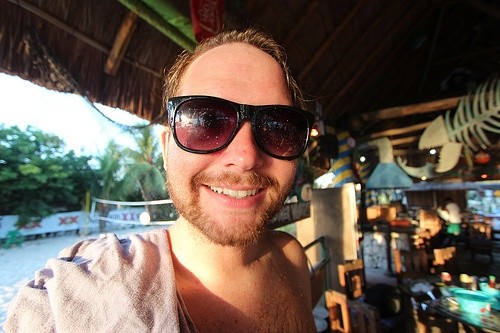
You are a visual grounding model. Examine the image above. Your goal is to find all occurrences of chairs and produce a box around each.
[324,289,376,333]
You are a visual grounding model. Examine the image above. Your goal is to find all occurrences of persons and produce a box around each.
[0,30,317,333]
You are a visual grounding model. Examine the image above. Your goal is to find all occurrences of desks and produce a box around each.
[411,277,500,333]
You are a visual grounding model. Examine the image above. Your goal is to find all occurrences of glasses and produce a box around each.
[166,95,317,161]
